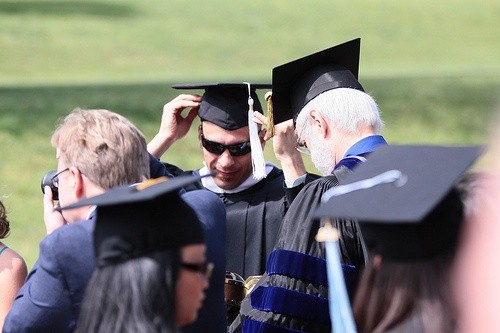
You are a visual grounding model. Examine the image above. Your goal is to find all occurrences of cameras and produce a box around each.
[42,170,60,200]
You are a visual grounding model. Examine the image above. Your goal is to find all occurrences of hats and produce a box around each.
[171,82,274,180]
[271,37,366,130]
[310,143,488,332]
[54,172,216,269]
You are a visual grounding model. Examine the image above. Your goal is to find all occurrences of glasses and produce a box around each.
[181,258,212,276]
[200,125,262,157]
[51,168,70,188]
[295,115,311,156]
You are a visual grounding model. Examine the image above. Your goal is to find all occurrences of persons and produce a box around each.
[1,107,227,333]
[311,143,489,333]
[228,38,390,333]
[451,136,500,333]
[0,201,28,332]
[144,82,322,327]
[52,173,219,333]
